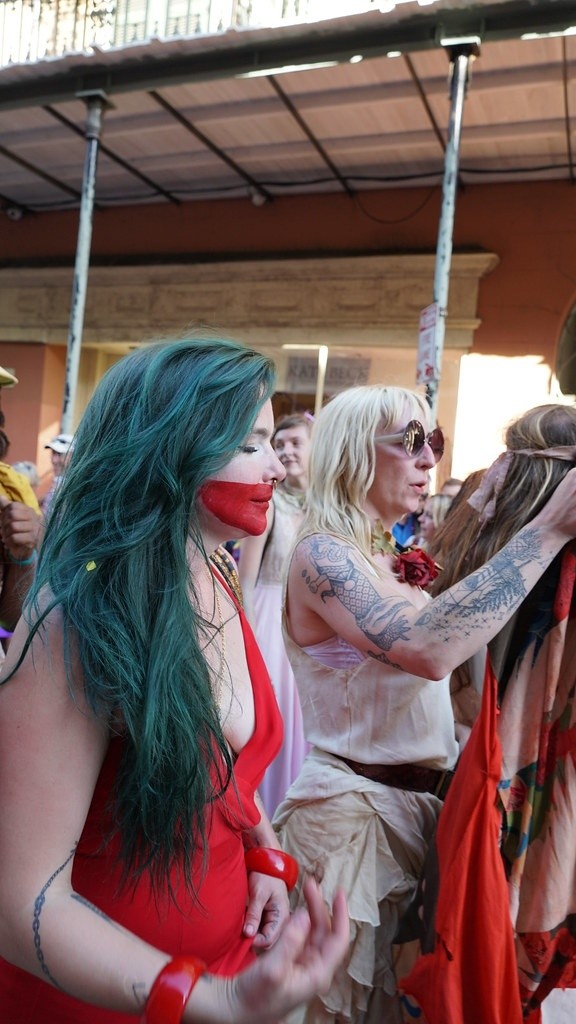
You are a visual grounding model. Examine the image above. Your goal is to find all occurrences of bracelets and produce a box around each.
[244,846,298,890]
[140,954,205,1024]
[10,547,36,565]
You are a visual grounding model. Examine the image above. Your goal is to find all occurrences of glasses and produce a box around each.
[374,419,444,464]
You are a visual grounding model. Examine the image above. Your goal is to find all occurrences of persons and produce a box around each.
[236,409,311,817]
[0,339,348,1024]
[277,384,576,1024]
[0,368,77,650]
[389,402,576,1024]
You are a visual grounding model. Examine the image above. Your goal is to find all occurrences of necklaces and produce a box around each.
[208,571,224,705]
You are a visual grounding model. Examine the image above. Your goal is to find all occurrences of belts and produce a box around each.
[328,753,455,802]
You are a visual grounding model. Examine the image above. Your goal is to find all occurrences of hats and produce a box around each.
[45,434,76,453]
[0,367,18,388]
